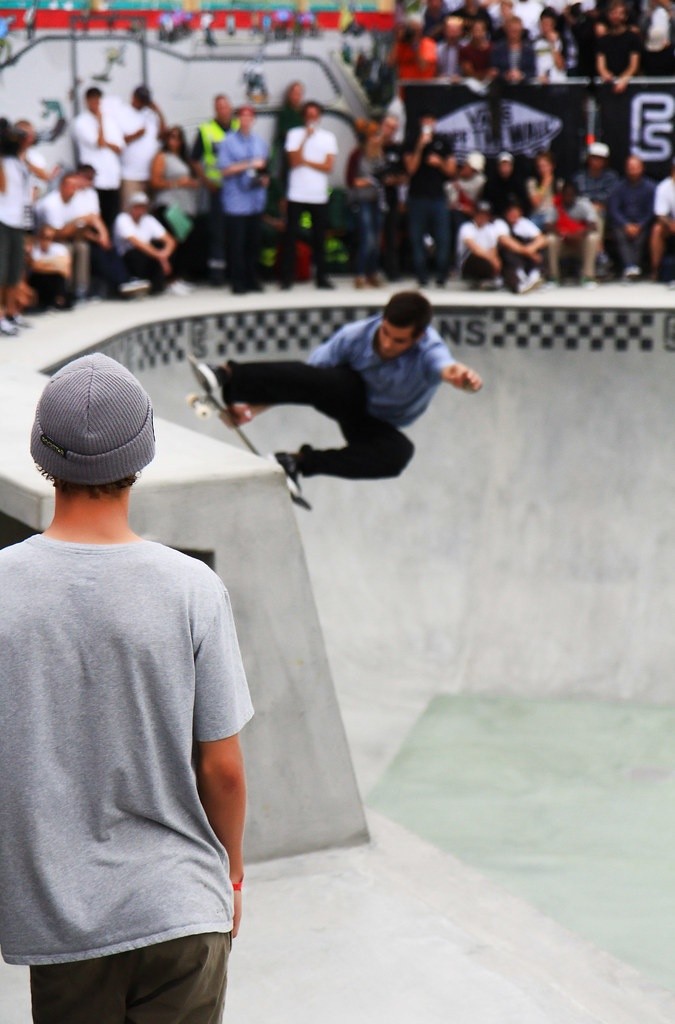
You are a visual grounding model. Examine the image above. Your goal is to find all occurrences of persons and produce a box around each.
[0,351,254,1024]
[344,116,675,295]
[200,291,482,496]
[355,0,674,143]
[0,80,338,336]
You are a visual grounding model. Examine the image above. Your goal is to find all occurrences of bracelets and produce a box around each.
[233,875,243,890]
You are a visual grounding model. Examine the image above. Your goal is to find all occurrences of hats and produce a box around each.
[467,151,485,171]
[29,353,156,485]
[587,142,610,157]
[128,192,150,204]
[498,150,512,161]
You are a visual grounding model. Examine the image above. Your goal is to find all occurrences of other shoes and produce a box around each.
[355,270,380,288]
[515,269,542,292]
[268,451,302,498]
[622,266,641,282]
[479,276,504,293]
[0,313,33,336]
[212,366,235,406]
[279,277,292,289]
[314,279,334,288]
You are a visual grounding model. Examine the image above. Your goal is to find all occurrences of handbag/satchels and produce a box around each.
[165,179,193,239]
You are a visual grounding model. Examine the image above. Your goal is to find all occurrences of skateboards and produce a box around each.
[186,352,313,511]
